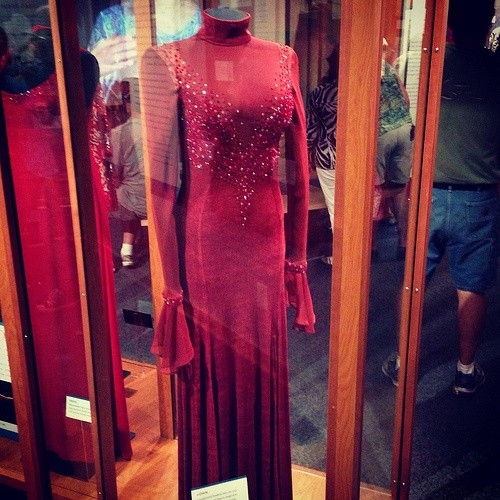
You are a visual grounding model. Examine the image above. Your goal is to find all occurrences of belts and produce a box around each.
[433,182,499,192]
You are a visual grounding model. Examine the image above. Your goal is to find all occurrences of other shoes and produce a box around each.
[396,245,407,261]
[372,246,379,258]
[120,242,136,267]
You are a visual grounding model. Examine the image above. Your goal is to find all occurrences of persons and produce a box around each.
[88,0,202,104]
[371,60,414,259]
[1,23,133,465]
[108,77,181,271]
[141,8,318,500]
[306,45,340,266]
[380,0,500,393]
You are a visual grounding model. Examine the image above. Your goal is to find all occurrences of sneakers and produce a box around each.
[321,255,333,266]
[453,364,486,396]
[382,351,401,386]
